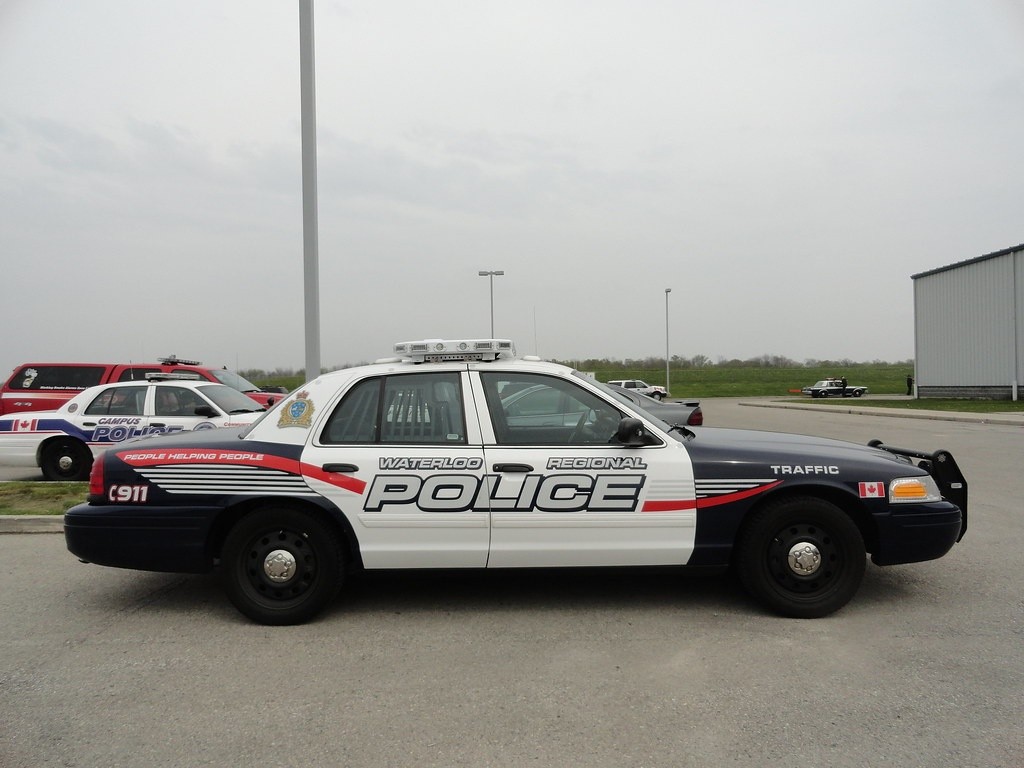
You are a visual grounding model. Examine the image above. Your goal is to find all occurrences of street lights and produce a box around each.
[478,271,504,338]
[664,287,673,399]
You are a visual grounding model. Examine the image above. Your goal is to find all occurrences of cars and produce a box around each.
[0,372,268,481]
[381,376,706,445]
[799,376,868,398]
[60,333,972,627]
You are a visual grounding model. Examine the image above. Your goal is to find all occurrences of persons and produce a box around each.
[906,374,912,395]
[842,376,848,398]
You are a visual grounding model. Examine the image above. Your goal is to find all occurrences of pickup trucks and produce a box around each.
[0,354,288,481]
